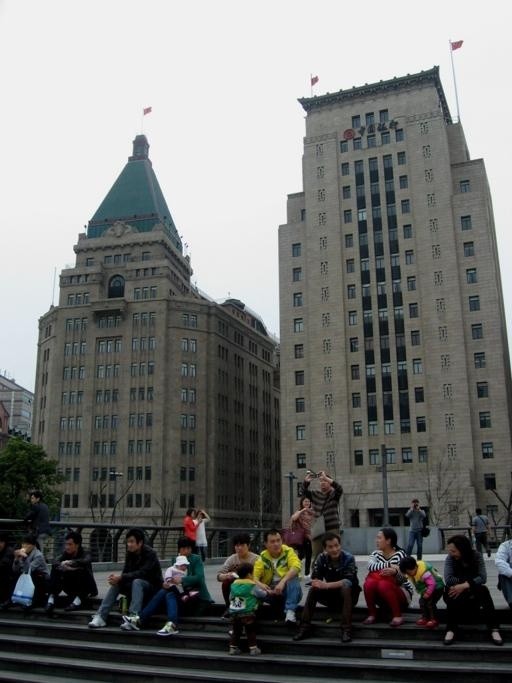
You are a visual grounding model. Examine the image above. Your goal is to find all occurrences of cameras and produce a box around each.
[414,503,418,508]
[314,474,320,478]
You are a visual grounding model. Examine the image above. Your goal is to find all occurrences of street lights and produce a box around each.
[109,470,124,561]
[284,471,298,518]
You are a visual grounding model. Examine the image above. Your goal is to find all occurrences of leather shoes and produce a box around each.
[444,633,454,645]
[340,627,351,642]
[491,632,503,645]
[65,601,84,611]
[46,602,54,611]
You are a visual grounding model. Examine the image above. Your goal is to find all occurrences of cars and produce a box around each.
[465,532,502,548]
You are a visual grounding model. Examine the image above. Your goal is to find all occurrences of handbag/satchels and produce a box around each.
[12,574,36,606]
[310,515,326,540]
[279,526,306,545]
[366,570,396,584]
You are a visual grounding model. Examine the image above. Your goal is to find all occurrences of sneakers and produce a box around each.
[88,613,108,627]
[416,618,438,629]
[182,591,198,601]
[364,616,377,624]
[119,614,143,631]
[390,616,404,626]
[157,621,180,636]
[221,610,311,656]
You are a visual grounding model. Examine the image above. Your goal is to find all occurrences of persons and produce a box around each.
[164,556,200,602]
[88,529,164,627]
[287,472,343,587]
[405,497,426,561]
[0,492,98,611]
[472,508,491,557]
[184,509,202,553]
[216,528,510,655]
[193,509,211,562]
[122,536,215,637]
